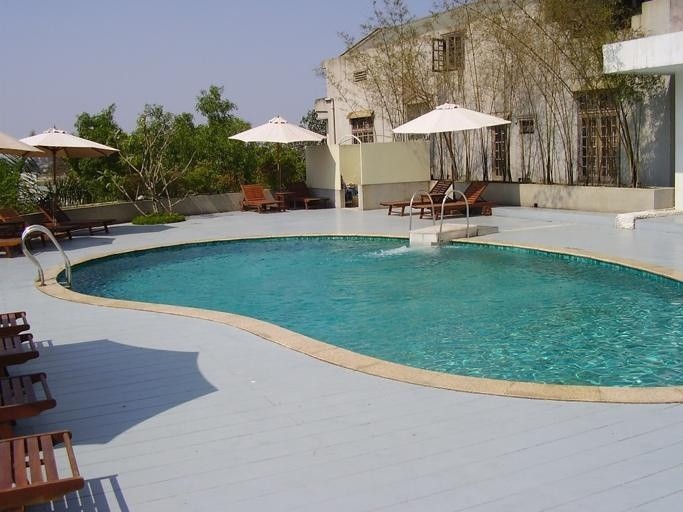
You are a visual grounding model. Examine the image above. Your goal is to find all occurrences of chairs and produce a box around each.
[0,333,40,377]
[0,198,117,257]
[0,311,30,336]
[239,183,285,214]
[286,180,330,210]
[0,428,84,512]
[411,180,496,220]
[380,180,453,217]
[0,372,57,440]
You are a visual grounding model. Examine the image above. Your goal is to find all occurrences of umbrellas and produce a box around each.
[18,125,120,217]
[226,114,328,189]
[0,130,48,157]
[388,102,511,198]
